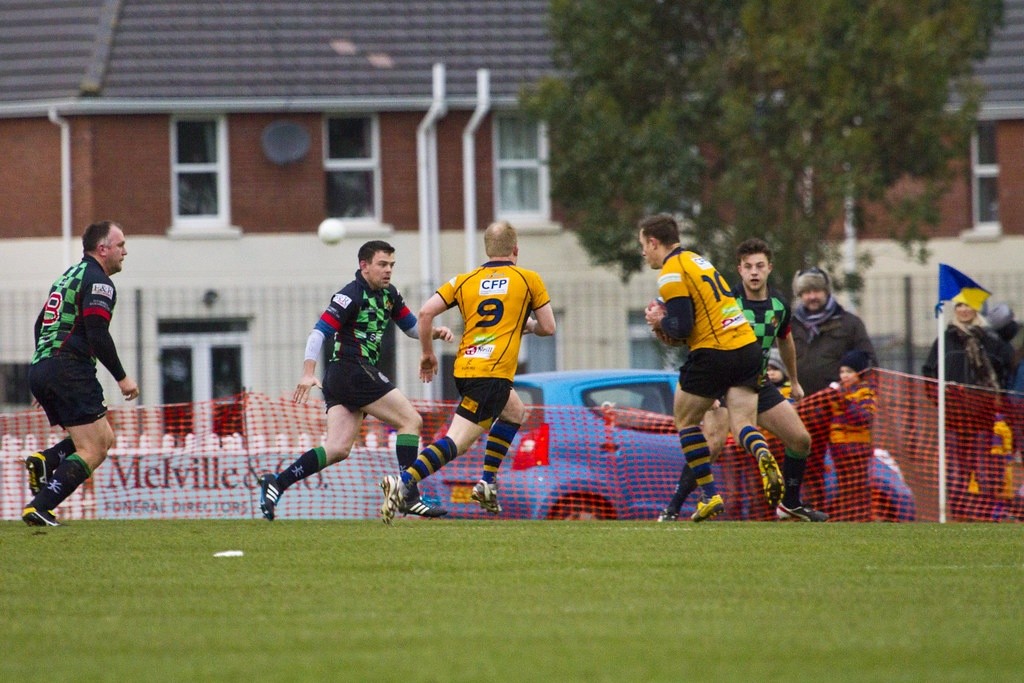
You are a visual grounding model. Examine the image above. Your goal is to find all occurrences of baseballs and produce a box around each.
[318,218,347,246]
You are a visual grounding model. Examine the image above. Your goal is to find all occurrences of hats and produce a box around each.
[837,349,869,380]
[792,266,833,297]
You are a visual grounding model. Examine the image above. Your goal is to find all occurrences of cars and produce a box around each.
[422,370,916,524]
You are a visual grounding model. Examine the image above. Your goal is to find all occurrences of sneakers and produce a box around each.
[691,495,725,523]
[471,480,503,514]
[380,474,409,524]
[658,510,679,522]
[777,500,830,523]
[26,453,52,496]
[758,451,786,510]
[257,473,282,522]
[22,504,63,528]
[399,498,449,518]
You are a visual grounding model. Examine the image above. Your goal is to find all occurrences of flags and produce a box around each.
[939,264,991,309]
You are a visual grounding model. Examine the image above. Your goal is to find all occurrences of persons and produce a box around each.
[260,241,447,521]
[988,304,1024,397]
[766,346,792,404]
[658,239,829,522]
[640,212,786,522]
[923,303,1013,521]
[829,349,878,522]
[22,220,140,527]
[789,265,878,522]
[379,222,558,525]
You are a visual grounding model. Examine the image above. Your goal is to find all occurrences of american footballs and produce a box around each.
[648,296,686,347]
